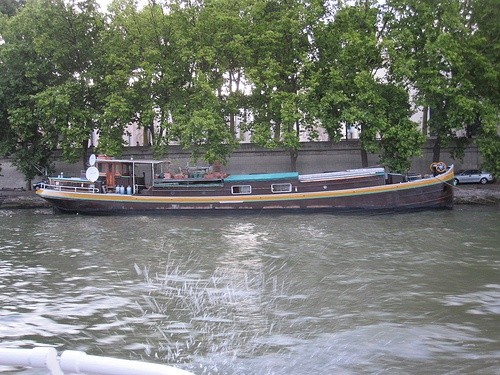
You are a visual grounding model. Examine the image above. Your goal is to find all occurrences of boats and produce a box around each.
[35,154,455,218]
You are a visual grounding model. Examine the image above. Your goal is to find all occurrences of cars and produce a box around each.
[454,167,493,185]
